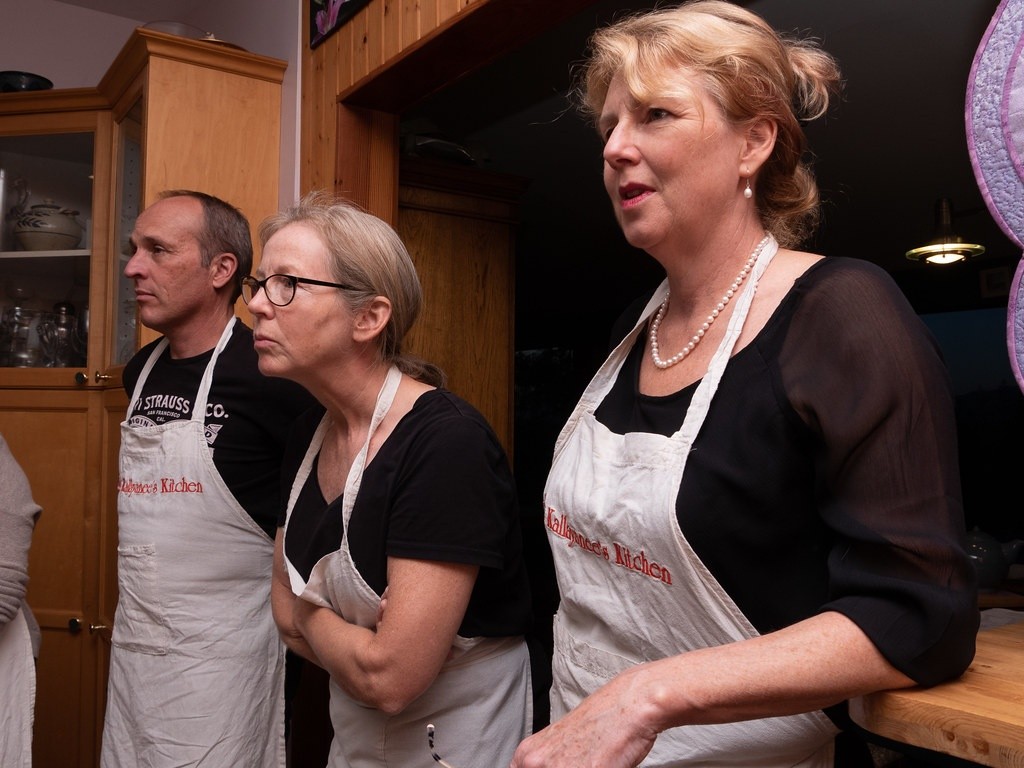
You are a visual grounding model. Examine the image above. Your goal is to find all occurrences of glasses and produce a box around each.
[238,274,355,307]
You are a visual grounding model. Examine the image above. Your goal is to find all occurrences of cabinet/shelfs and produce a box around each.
[0,27,287,768]
[397,134,524,481]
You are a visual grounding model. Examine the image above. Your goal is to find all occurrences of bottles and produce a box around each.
[14,199,83,250]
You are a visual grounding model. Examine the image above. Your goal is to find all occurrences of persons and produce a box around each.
[508,0,984,768]
[101,190,328,768]
[247,202,535,768]
[0,433,44,768]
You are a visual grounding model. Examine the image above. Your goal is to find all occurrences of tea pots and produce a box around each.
[959,526,1023,593]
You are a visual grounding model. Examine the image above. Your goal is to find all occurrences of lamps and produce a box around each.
[905,196,986,271]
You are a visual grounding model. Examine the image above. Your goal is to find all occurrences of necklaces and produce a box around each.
[650,230,771,369]
